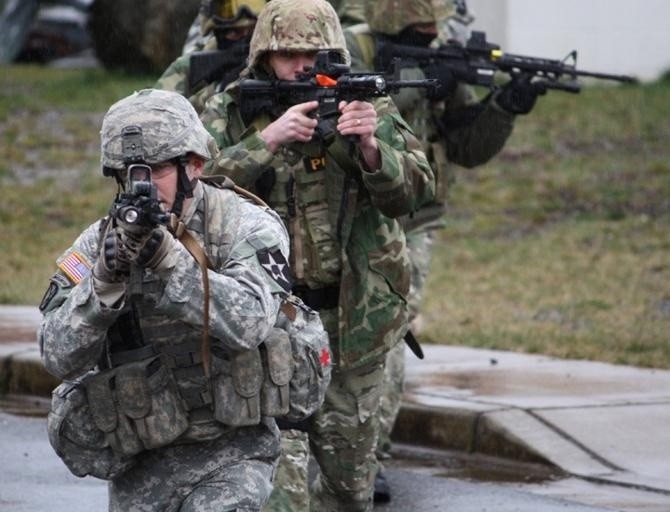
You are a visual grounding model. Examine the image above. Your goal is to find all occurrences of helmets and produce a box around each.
[99,89,218,170]
[198,0,273,39]
[247,0,352,69]
[363,1,473,36]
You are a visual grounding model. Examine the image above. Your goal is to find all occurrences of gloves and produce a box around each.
[494,71,548,116]
[422,60,479,102]
[133,229,166,266]
[91,229,127,285]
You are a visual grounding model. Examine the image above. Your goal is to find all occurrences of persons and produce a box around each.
[158,0,269,113]
[336,0,552,499]
[197,0,437,512]
[37,87,297,512]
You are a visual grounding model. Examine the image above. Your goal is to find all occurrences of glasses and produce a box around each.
[210,0,269,26]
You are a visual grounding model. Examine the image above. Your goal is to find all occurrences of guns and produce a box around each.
[241,51,443,142]
[189,42,250,97]
[110,164,168,298]
[375,22,639,100]
